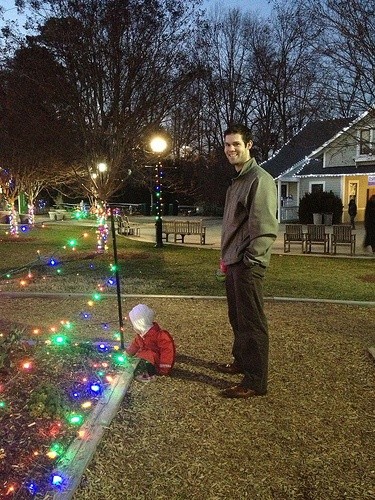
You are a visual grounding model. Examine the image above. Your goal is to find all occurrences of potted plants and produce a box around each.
[303,189,335,223]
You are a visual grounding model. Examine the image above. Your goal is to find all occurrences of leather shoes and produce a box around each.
[222,383,267,398]
[216,361,242,373]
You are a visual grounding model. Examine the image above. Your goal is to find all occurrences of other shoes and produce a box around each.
[136,372,156,382]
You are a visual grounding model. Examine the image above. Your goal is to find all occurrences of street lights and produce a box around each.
[98,163,107,185]
[149,137,168,248]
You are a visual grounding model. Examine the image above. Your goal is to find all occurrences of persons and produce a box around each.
[123,305,175,380]
[217,123,277,397]
[348,196,357,229]
[362,194,375,252]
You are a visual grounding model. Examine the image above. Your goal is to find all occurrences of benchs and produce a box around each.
[161,219,206,246]
[115,214,140,235]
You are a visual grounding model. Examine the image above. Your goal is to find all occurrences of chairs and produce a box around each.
[282,224,306,254]
[331,225,356,256]
[304,225,330,254]
